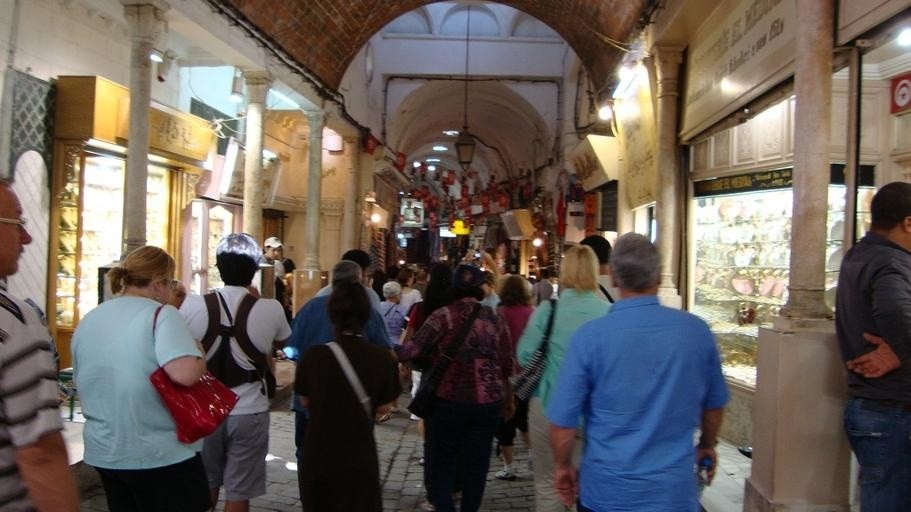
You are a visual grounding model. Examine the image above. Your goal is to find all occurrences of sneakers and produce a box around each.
[495,471,515,480]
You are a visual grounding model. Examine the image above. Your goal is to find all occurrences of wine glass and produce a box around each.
[694,301,783,368]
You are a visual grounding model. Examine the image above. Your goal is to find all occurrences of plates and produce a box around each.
[695,190,846,298]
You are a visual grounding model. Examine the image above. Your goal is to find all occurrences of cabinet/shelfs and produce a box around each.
[51,147,184,337]
[689,173,875,391]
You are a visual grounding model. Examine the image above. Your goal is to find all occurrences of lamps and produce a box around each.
[230,66,246,104]
[453,4,480,173]
[148,32,178,85]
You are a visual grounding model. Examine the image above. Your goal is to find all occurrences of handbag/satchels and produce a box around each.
[151,358,240,444]
[407,371,437,418]
[508,348,546,403]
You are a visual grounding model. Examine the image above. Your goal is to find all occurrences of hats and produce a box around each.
[264,237,283,249]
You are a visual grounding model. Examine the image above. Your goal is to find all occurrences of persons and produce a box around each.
[0,175,81,512]
[835,182,911,512]
[71,245,214,512]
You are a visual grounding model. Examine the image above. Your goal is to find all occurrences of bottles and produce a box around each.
[695,461,709,500]
